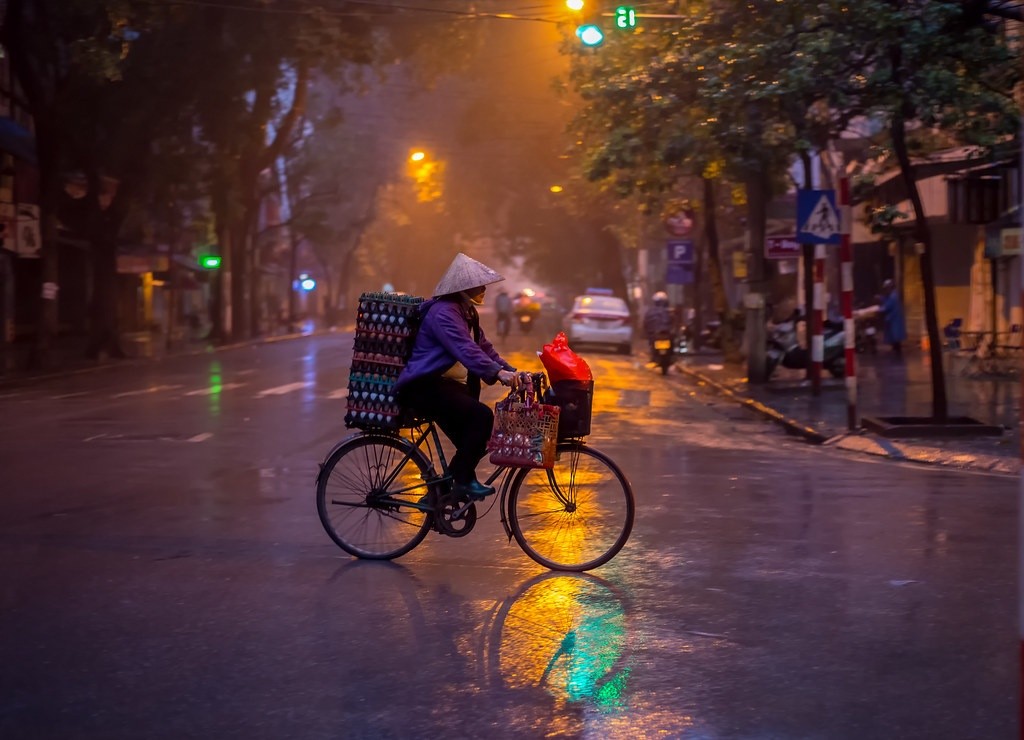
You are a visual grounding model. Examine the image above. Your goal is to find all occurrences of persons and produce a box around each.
[495,286,512,335]
[513,291,541,332]
[391,252,523,515]
[873,279,908,366]
[642,290,680,374]
[944,318,963,350]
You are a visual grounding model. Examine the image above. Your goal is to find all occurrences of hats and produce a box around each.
[431,252,505,297]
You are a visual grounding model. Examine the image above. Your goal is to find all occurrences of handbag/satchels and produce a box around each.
[540,331,592,386]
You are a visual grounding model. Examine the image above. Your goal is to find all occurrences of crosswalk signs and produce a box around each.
[793,189,846,245]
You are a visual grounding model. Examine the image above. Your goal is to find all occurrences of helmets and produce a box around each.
[652,292,667,300]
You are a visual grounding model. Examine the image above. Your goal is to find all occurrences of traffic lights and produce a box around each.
[614,6,637,31]
[580,25,605,48]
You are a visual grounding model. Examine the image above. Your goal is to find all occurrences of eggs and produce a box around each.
[349,303,414,422]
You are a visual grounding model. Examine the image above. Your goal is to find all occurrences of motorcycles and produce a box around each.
[764,303,861,379]
[646,319,678,375]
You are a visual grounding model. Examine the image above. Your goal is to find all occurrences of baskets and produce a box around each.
[490,372,560,469]
[542,380,594,438]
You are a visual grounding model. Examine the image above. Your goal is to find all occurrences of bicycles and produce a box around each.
[316,370,636,572]
[496,307,510,345]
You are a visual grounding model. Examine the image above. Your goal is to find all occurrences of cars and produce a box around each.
[560,293,633,355]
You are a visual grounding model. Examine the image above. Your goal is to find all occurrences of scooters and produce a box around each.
[517,303,538,334]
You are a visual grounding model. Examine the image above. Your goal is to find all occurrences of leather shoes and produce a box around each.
[419,492,433,512]
[452,473,495,497]
